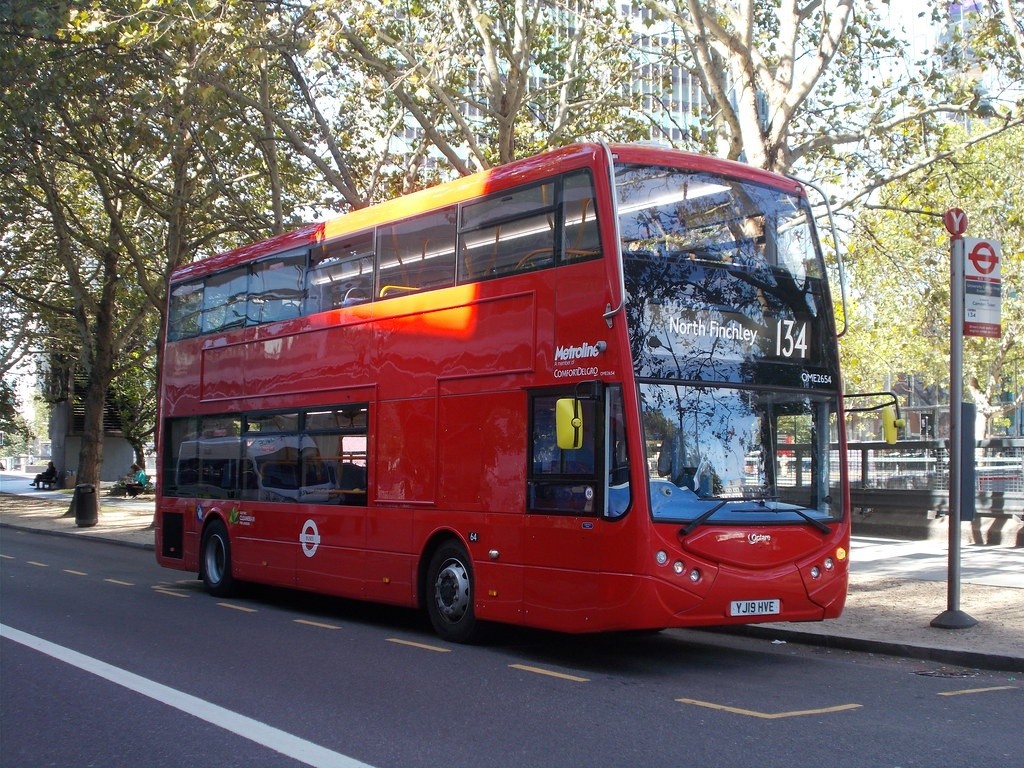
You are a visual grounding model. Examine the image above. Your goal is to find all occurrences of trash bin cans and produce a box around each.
[74,483,98,527]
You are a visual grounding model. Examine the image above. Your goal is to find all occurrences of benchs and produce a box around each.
[174,458,365,501]
[40,471,60,490]
[121,476,151,498]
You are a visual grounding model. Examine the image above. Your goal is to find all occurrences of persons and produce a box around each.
[657,409,724,499]
[29,462,56,490]
[126,465,146,500]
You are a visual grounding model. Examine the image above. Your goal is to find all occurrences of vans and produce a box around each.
[173,430,349,510]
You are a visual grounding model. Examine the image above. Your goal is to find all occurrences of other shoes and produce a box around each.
[132,496,136,499]
[29,483,35,486]
[35,487,40,490]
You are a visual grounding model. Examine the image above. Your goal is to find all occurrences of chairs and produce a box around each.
[276,284,419,320]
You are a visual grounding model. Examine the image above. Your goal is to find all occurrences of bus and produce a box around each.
[153,140,907,650]
